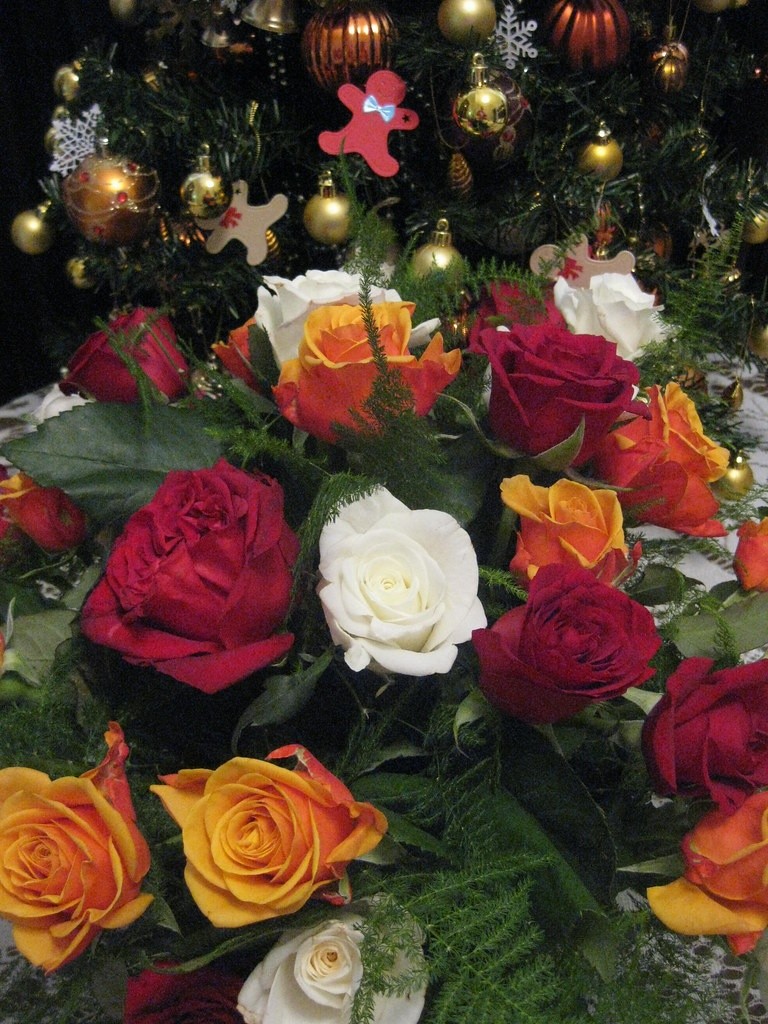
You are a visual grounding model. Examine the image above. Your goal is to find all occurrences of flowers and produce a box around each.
[0,243,768,1024]
